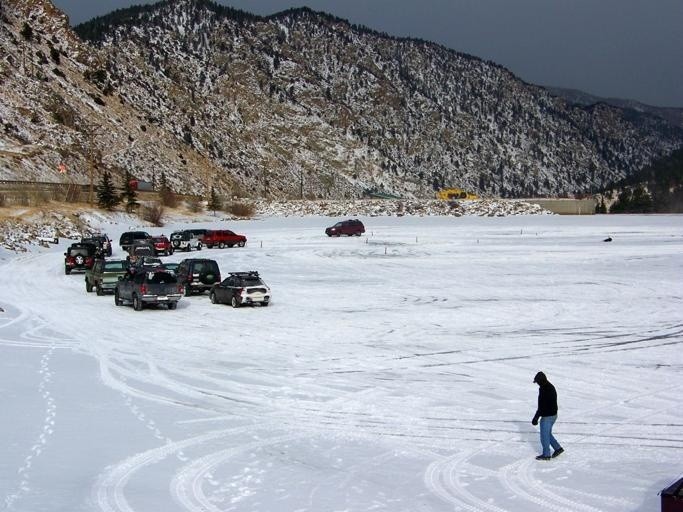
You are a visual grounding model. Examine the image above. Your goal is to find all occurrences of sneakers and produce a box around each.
[536,454,551,461]
[552,447,564,458]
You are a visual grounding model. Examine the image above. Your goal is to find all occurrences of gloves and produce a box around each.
[532,417,538,425]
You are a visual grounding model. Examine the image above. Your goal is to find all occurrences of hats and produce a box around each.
[533,372,545,382]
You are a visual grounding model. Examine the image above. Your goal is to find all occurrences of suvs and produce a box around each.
[209,271,271,308]
[175,258,221,297]
[326,218,366,237]
[114,268,185,312]
[63,228,248,296]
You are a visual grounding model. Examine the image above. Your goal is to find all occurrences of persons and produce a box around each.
[531,370,564,461]
